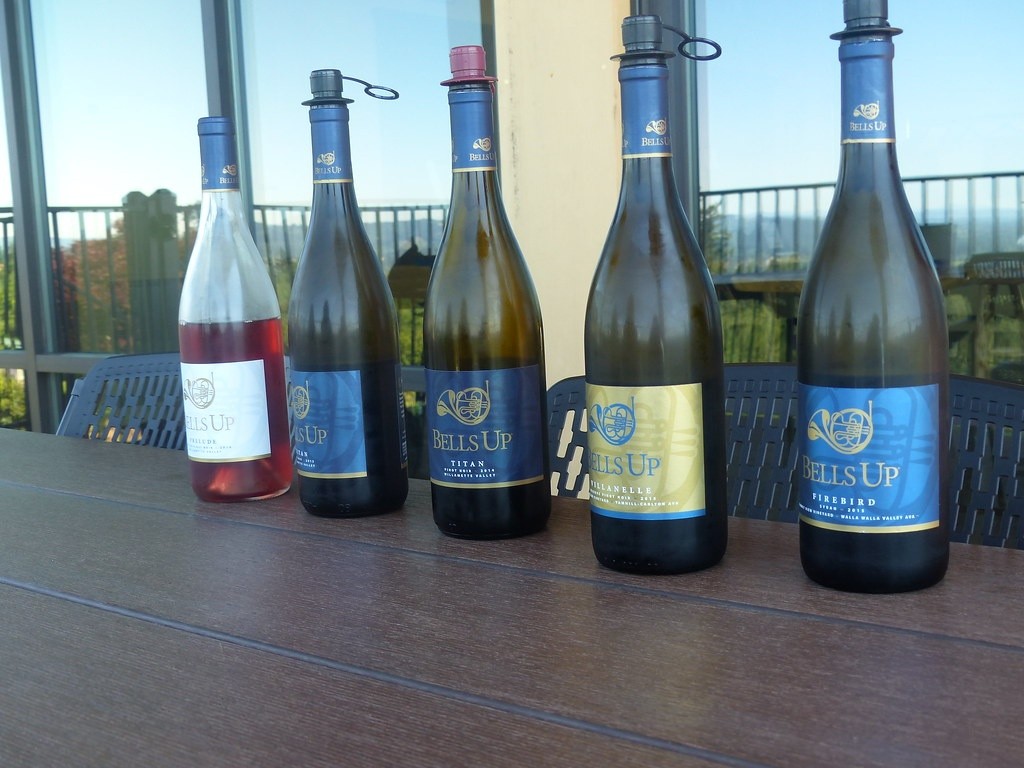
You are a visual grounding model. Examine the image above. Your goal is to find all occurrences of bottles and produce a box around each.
[796,1,949,593]
[178,116,293,505]
[288,70,409,518]
[423,46,552,541]
[585,15,729,574]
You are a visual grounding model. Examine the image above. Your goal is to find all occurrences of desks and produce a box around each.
[1,427,1024,767]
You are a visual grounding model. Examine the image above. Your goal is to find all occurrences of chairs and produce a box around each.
[53,357,1024,546]
